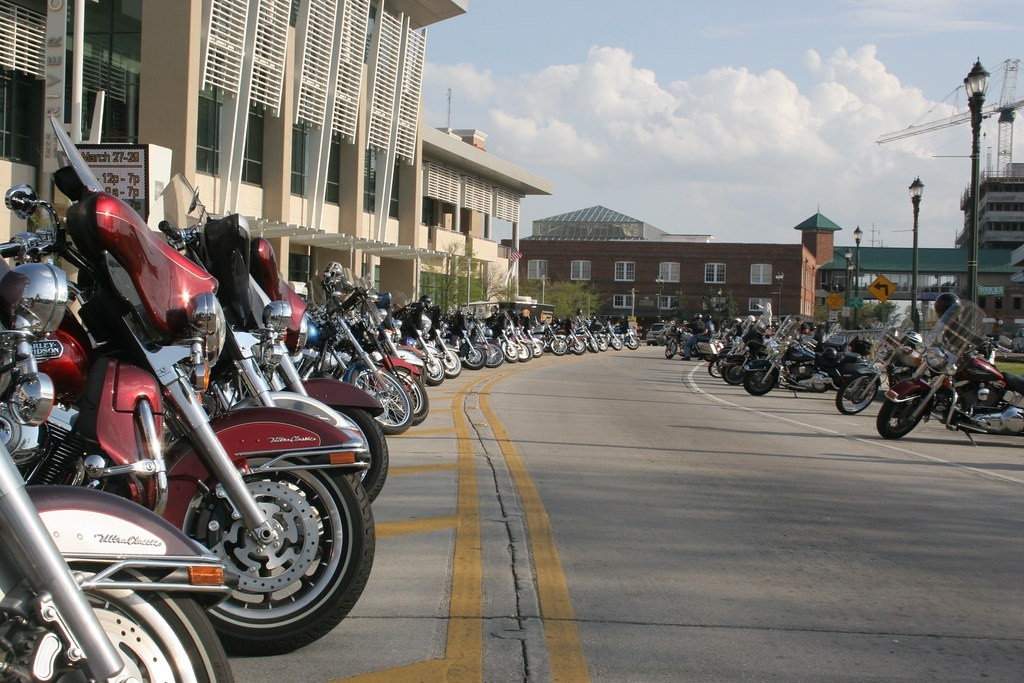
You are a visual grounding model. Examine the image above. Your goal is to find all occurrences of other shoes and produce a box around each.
[681,356,690,361]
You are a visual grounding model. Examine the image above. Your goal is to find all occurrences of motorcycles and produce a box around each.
[662,297,1024,447]
[0,111,642,683]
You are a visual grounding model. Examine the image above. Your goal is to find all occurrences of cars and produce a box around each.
[646,323,667,346]
[1012,327,1024,353]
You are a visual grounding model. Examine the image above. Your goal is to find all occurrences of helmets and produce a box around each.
[0,263,68,336]
[734,317,742,322]
[705,314,712,320]
[906,331,922,349]
[419,295,431,308]
[935,293,961,319]
[508,309,515,318]
[693,313,702,319]
[823,347,837,359]
[748,315,755,322]
[577,310,582,315]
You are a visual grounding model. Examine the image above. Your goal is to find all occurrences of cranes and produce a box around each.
[875,58,1024,147]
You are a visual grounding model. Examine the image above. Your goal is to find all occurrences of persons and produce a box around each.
[725,315,755,341]
[680,313,714,361]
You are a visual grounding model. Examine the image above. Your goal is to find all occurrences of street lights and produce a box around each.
[854,225,862,329]
[717,287,723,328]
[776,271,785,317]
[907,176,924,327]
[962,56,992,332]
[655,275,665,323]
